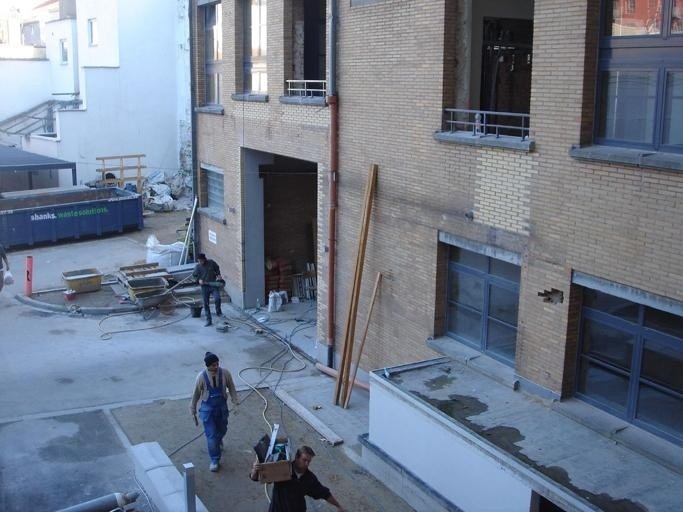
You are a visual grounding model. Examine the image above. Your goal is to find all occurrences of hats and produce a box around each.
[204,352,219,367]
[195,254,206,259]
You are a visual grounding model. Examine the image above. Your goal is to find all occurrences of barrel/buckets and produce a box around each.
[189,304,203,317]
[64,289,77,300]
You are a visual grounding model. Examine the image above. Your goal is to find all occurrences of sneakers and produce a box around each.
[210,462,219,472]
[204,320,211,327]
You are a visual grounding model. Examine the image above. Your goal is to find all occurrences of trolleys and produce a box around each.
[133,288,173,321]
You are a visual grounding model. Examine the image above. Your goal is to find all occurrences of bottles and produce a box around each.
[256,298,260,314]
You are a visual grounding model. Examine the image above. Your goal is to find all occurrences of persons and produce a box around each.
[190,352,241,471]
[0,244,9,292]
[193,254,225,327]
[249,446,347,512]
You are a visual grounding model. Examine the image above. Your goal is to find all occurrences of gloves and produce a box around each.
[192,414,198,426]
